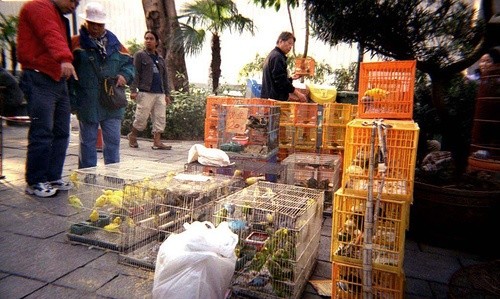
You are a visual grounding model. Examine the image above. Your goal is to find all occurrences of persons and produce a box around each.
[260,32,306,102]
[479,48,500,76]
[16,0,80,196]
[69,4,135,184]
[128,30,172,149]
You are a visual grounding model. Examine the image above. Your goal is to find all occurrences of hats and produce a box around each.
[77,3,109,24]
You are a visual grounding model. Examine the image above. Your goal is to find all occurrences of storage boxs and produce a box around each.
[204,59,419,299]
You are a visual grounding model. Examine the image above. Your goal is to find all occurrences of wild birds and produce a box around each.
[71,172,79,188]
[67,195,83,213]
[233,169,244,177]
[365,88,389,100]
[349,204,395,232]
[339,273,390,299]
[215,185,314,275]
[245,175,265,184]
[89,172,233,231]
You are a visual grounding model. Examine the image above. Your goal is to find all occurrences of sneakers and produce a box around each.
[24,182,60,197]
[48,180,75,190]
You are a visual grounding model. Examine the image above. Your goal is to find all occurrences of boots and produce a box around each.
[127,127,139,148]
[152,132,172,150]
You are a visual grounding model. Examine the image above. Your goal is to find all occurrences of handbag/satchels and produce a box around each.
[99,75,129,110]
